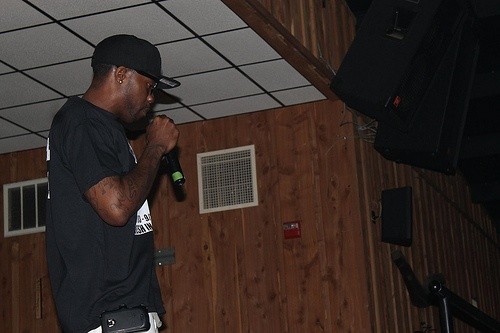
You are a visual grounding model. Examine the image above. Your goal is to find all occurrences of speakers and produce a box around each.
[381,186,413,247]
[328,0,480,175]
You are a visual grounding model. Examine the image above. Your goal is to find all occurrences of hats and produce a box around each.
[91,35,181,90]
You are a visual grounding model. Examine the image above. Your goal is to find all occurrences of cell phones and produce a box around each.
[101,308,150,333]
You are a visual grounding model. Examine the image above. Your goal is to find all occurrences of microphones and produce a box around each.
[149,117,186,187]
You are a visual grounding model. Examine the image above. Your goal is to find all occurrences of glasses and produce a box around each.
[126,68,158,92]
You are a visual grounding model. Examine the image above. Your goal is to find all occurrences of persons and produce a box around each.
[44,32,180,333]
